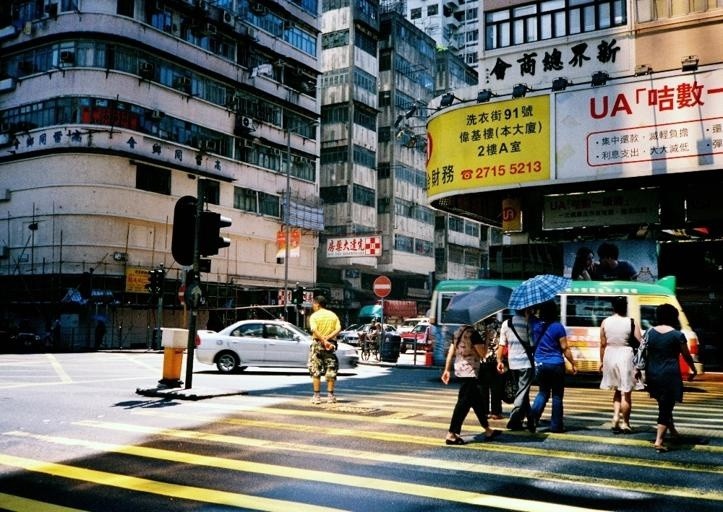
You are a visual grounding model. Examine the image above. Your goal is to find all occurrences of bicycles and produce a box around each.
[360,334,380,362]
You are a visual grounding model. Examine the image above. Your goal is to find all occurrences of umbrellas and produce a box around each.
[443,285,513,327]
[508,274,569,311]
[358,304,382,321]
[92,315,108,322]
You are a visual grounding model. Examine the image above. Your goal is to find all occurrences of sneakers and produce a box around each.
[506,408,522,430]
[445,438,465,446]
[327,394,337,404]
[654,445,670,453]
[610,426,634,434]
[310,394,321,405]
[484,429,502,440]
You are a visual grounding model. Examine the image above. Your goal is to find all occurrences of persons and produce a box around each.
[369,320,384,340]
[642,303,697,453]
[595,242,637,281]
[597,296,642,433]
[572,247,598,279]
[94,321,105,350]
[441,300,577,445]
[307,296,342,403]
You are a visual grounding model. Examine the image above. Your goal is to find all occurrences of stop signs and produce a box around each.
[372,275,391,298]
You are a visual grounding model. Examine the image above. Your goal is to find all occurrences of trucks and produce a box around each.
[357,299,418,327]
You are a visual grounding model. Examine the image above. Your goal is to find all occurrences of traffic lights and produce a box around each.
[199,208,234,257]
[147,270,154,292]
[291,289,297,304]
[297,285,306,305]
[153,268,163,292]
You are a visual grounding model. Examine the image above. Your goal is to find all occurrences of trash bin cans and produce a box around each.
[381,342,399,362]
[152,328,164,350]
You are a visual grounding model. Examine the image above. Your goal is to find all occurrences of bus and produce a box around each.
[426,272,706,391]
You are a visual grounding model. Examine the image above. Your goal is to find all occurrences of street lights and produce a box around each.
[281,118,321,321]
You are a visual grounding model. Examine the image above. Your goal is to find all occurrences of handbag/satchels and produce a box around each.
[627,317,641,349]
[632,326,653,370]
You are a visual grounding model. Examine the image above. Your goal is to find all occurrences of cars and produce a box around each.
[193,317,359,376]
[336,318,431,355]
[9,332,40,352]
